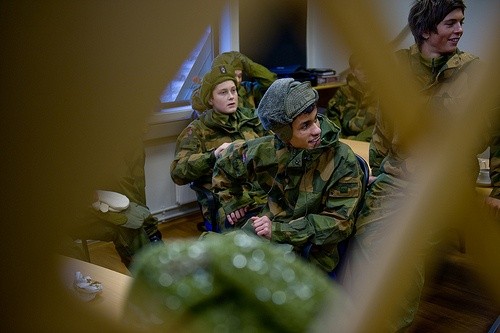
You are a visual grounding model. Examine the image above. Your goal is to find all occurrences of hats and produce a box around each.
[200,63,240,106]
[256,78,319,132]
[212,50,244,71]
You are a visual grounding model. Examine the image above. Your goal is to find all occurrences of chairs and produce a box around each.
[190,152,369,280]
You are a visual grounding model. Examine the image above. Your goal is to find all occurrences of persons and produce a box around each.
[70,148,163,277]
[327,52,378,142]
[170,64,273,236]
[213,78,365,274]
[339,0,500,333]
[191,50,279,116]
